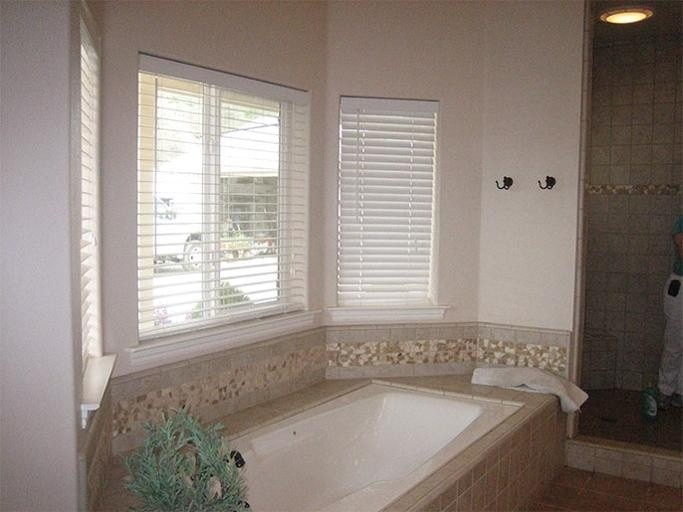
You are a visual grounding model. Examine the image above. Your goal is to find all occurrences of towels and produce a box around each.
[469,364,590,417]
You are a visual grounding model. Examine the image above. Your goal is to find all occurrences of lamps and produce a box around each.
[591,0,657,28]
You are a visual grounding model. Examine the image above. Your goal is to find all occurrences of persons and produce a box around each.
[657,215,682,411]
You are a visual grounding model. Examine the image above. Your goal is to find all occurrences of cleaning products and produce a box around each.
[640,381,660,422]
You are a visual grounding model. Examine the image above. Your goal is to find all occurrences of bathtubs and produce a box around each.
[92,373,569,512]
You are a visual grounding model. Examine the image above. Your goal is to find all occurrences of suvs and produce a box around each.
[151,192,206,270]
